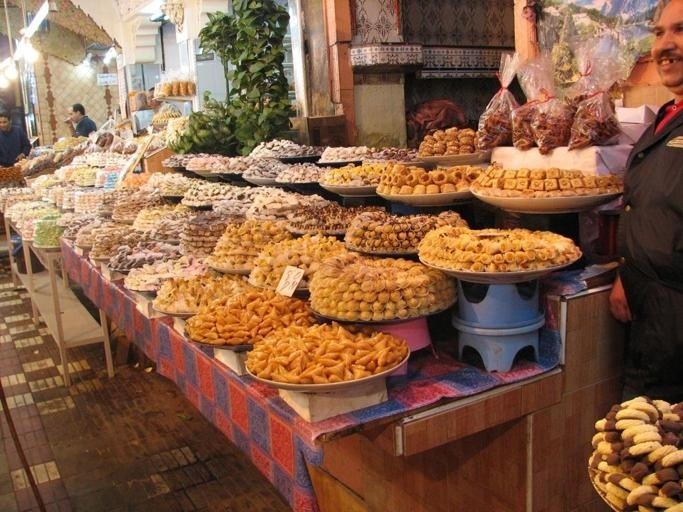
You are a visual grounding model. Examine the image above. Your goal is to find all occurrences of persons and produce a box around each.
[0,112,33,169]
[606,0,683,407]
[145,87,161,111]
[63,103,96,139]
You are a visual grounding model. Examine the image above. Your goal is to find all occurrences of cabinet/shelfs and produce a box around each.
[1,139,626,511]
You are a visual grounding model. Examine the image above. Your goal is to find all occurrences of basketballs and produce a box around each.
[587,393,683,512]
